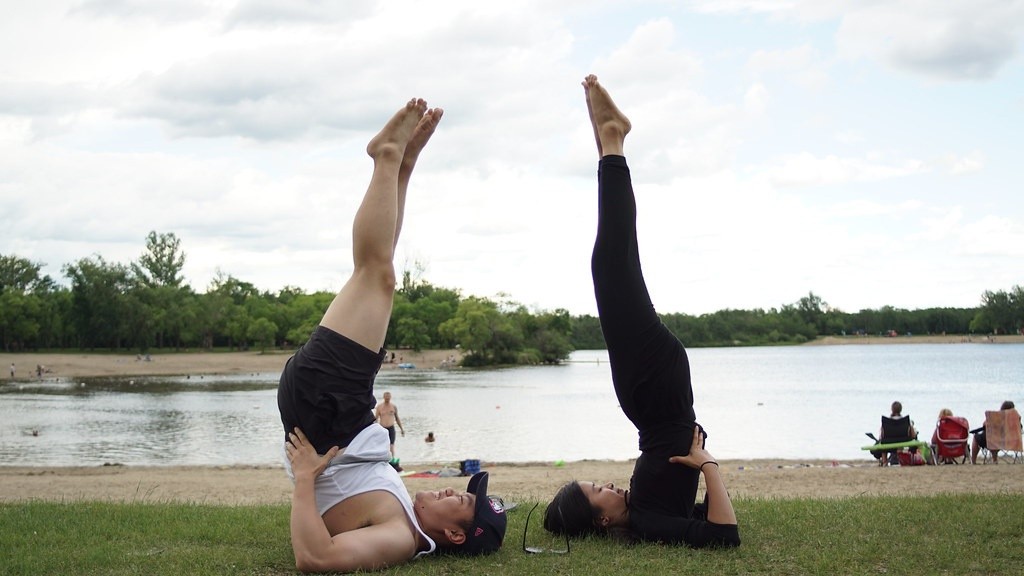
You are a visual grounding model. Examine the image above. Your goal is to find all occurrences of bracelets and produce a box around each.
[700,461,719,471]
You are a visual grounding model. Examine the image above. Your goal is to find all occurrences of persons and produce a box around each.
[276,98,507,573]
[971,401,1023,464]
[882,400,918,467]
[931,408,954,464]
[10,363,16,377]
[542,74,741,550]
[36,364,42,376]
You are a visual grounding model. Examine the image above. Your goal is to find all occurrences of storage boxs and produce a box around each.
[460,459,479,475]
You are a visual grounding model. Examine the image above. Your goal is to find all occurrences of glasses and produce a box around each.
[522,502,571,555]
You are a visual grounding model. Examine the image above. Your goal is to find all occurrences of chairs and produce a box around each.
[880,410,1024,467]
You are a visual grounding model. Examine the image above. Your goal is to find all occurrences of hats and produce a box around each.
[459,470,507,557]
